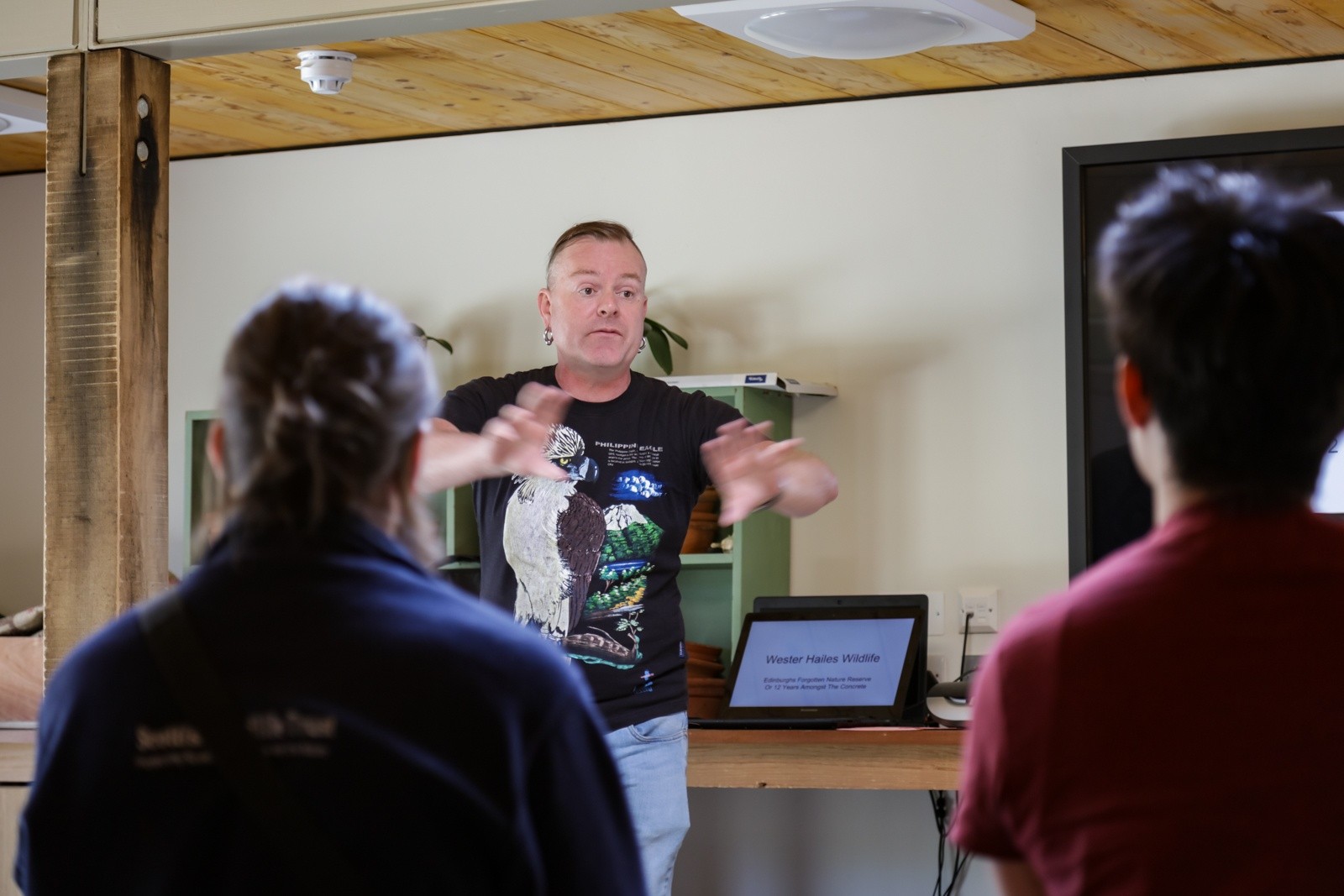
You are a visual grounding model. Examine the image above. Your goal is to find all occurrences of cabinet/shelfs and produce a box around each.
[443,386,796,688]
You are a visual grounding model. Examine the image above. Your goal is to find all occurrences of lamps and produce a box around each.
[672,0,1039,62]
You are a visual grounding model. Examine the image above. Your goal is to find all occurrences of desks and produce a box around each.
[681,708,985,896]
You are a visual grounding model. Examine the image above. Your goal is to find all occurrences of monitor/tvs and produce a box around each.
[1062,125,1344,590]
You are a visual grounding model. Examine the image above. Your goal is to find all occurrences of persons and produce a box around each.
[13,283,651,896]
[949,160,1344,896]
[413,224,840,896]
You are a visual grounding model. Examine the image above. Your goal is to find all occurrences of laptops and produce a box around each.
[686,595,931,730]
